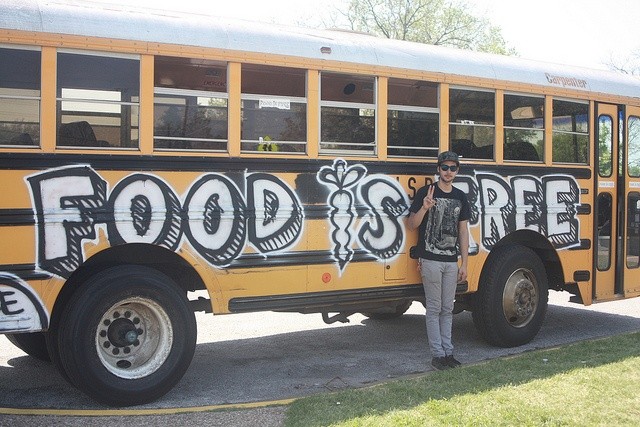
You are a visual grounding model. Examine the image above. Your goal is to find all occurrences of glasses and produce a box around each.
[440,165,459,172]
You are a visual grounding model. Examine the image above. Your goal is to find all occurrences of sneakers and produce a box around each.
[445,355,461,368]
[431,357,452,372]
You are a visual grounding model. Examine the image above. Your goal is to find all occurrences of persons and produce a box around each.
[408,150,470,372]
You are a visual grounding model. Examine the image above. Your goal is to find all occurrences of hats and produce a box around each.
[437,151,459,164]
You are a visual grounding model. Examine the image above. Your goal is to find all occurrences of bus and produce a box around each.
[0,0,640,406]
